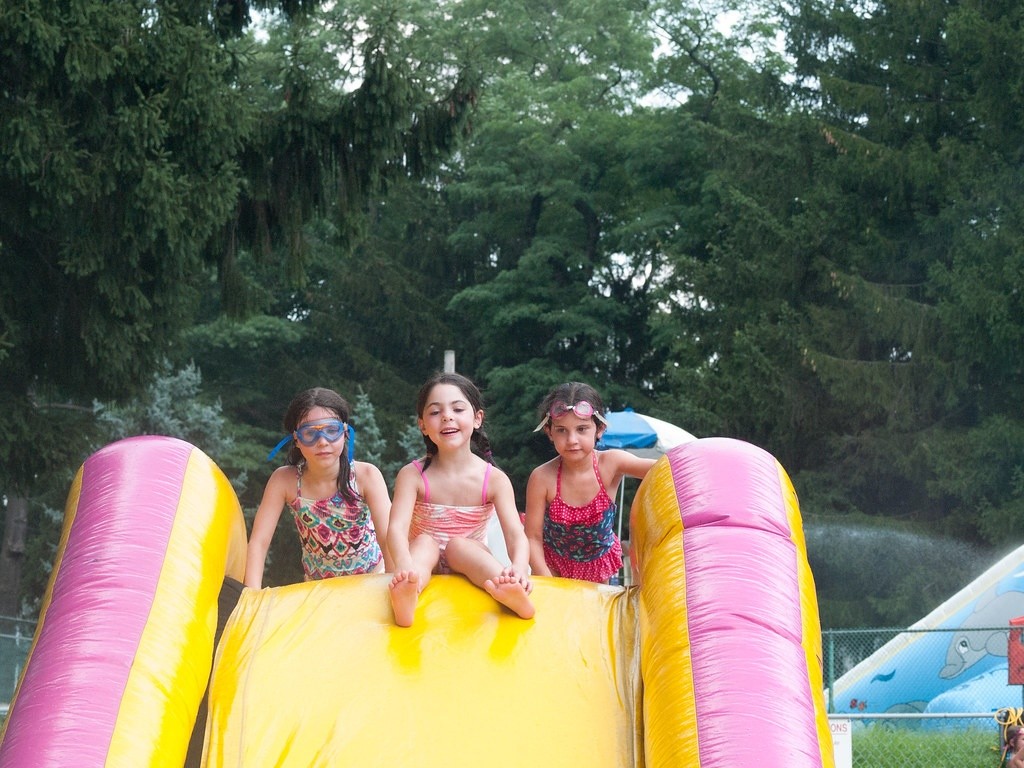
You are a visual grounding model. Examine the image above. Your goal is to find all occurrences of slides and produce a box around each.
[196,573,639,766]
[824,541,1024,732]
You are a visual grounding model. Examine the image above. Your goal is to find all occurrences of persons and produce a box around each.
[385,372,543,626]
[241,389,392,591]
[1002,725,1024,768]
[525,381,658,588]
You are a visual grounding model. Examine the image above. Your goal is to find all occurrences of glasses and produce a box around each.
[547,401,608,430]
[295,418,344,447]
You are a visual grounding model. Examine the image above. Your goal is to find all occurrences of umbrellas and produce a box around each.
[596,407,699,590]
